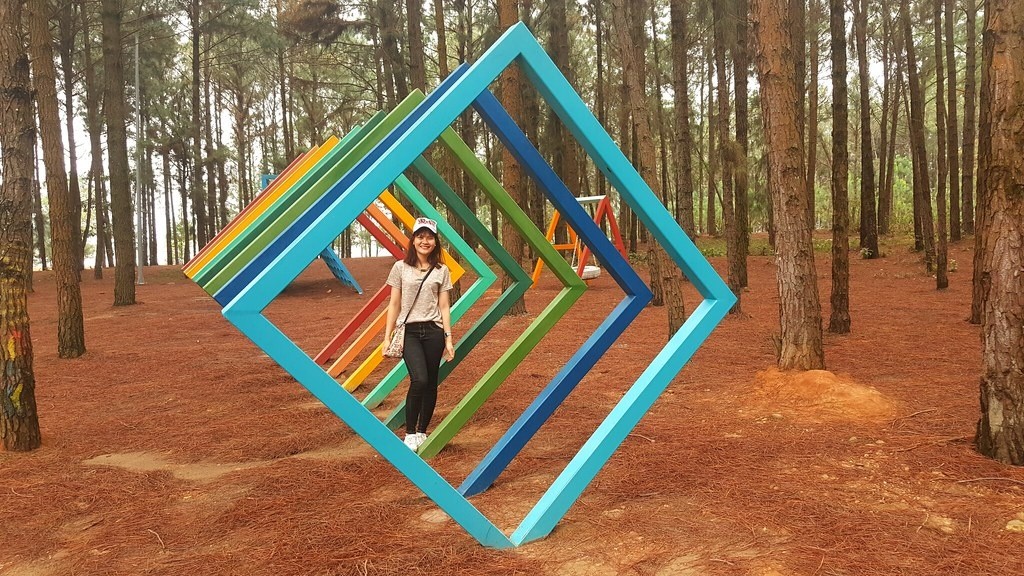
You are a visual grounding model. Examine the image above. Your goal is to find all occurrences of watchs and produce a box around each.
[444,333,451,337]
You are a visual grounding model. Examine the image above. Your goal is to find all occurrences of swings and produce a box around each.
[570,203,603,281]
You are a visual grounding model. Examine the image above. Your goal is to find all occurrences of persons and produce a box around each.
[383,217,455,453]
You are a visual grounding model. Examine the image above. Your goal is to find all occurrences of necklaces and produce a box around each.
[415,265,430,272]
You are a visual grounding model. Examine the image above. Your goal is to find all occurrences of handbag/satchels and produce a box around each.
[384,323,405,363]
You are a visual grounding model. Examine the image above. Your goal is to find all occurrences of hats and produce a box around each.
[413,217,438,235]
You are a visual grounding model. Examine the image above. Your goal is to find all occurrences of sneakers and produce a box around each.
[404,432,426,452]
